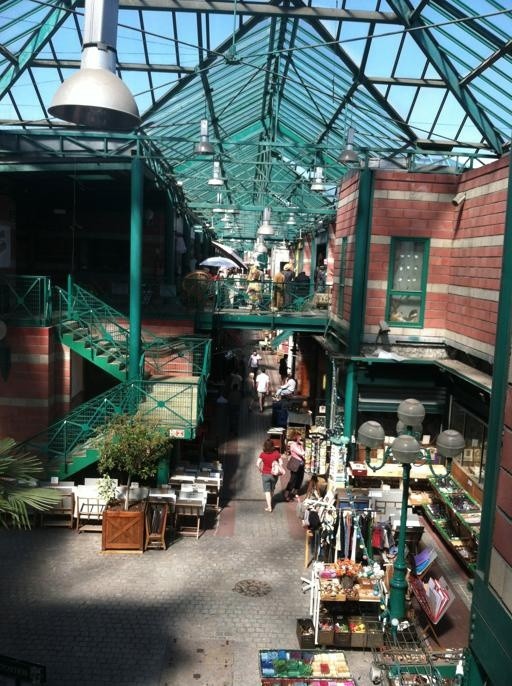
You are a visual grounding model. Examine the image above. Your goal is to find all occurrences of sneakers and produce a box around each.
[256,403,264,413]
[248,406,253,413]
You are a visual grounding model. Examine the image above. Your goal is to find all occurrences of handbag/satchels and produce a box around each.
[286,456,302,473]
[270,460,286,477]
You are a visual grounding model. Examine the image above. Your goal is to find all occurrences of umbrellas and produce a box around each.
[198,254,239,270]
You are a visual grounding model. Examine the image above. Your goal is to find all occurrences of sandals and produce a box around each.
[282,489,299,503]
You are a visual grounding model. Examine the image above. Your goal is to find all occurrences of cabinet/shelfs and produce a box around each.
[421,475,481,575]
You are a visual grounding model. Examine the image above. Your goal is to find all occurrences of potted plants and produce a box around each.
[91,412,172,551]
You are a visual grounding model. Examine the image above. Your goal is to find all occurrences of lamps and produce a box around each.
[257,235,267,252]
[337,126,358,163]
[214,193,241,234]
[208,162,224,186]
[193,97,216,155]
[257,210,274,235]
[310,167,325,190]
[286,214,296,225]
[48,0,142,130]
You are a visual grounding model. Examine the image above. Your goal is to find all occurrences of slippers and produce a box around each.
[263,502,274,512]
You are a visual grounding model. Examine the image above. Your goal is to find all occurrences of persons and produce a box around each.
[272,374,298,402]
[255,438,286,512]
[239,353,248,376]
[283,429,307,503]
[279,353,291,385]
[255,366,270,412]
[243,370,258,412]
[248,349,262,377]
[203,262,327,313]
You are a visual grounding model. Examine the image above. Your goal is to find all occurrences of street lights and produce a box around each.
[357,398,466,626]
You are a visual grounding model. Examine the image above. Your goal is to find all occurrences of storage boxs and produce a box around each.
[316,562,386,649]
